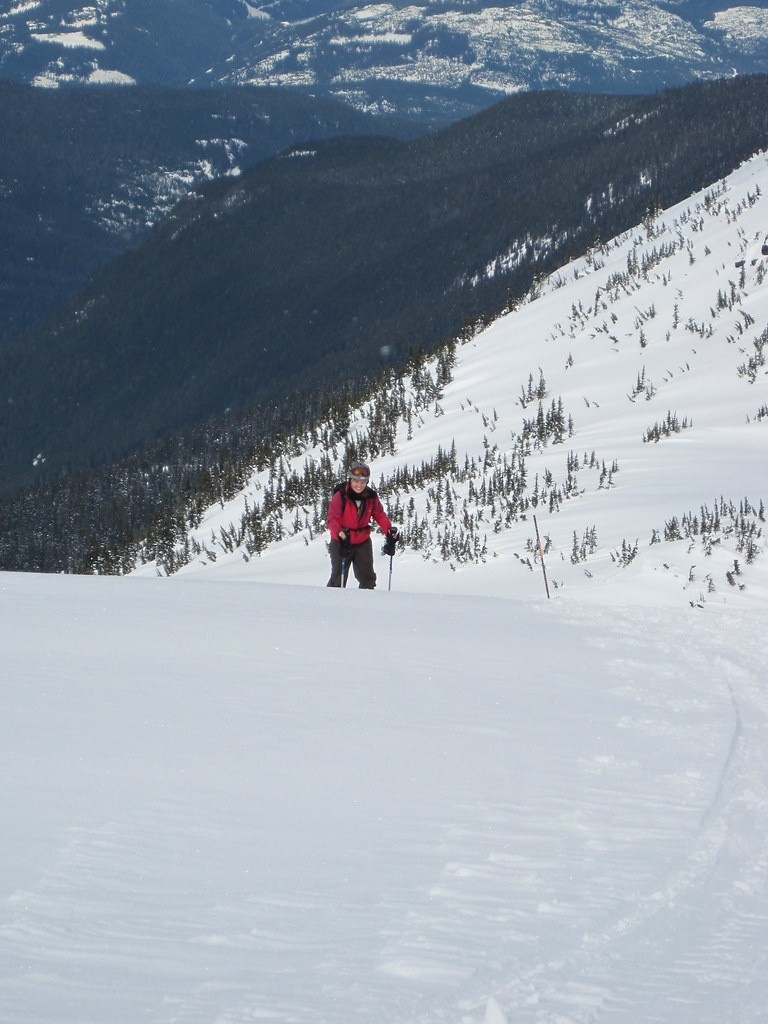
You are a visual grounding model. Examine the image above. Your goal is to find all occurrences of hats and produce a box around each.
[349,462,370,480]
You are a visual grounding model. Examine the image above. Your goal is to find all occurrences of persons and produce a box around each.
[326,461,401,590]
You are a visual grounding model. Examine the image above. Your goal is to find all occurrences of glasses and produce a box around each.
[352,467,369,476]
